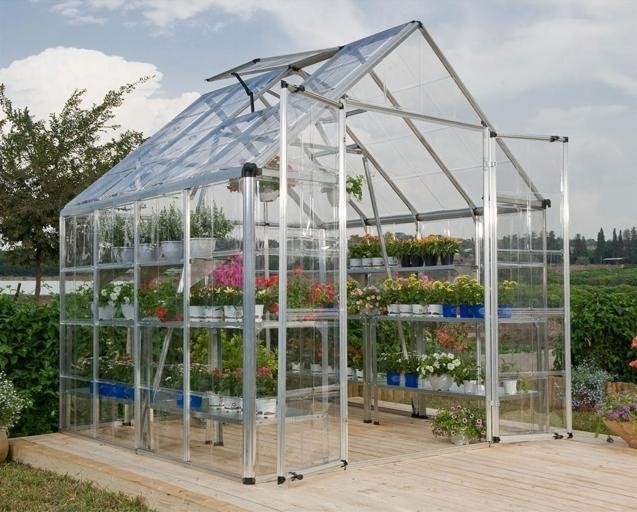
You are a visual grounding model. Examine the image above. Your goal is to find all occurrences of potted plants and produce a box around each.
[132,215,156,264]
[157,202,183,259]
[103,214,133,264]
[190,199,239,253]
[500,358,528,395]
[450,355,480,395]
[396,236,459,266]
[441,274,514,319]
[347,231,397,268]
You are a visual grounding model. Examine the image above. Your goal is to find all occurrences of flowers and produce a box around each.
[76,281,134,305]
[592,388,636,422]
[305,281,336,310]
[256,276,279,314]
[396,351,419,374]
[433,322,473,351]
[424,399,487,445]
[76,355,135,386]
[138,282,183,321]
[212,366,278,399]
[321,173,368,202]
[350,283,384,331]
[376,351,403,372]
[169,361,215,392]
[0,367,27,430]
[188,285,266,305]
[287,264,305,309]
[381,270,444,303]
[415,352,452,393]
[226,155,298,203]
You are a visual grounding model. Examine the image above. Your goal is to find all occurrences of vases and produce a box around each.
[90,380,134,402]
[208,395,277,419]
[98,305,117,320]
[461,379,477,395]
[176,391,203,410]
[387,370,400,386]
[364,308,377,315]
[260,190,281,202]
[189,305,266,323]
[404,372,418,387]
[602,416,636,449]
[0,429,9,464]
[451,429,466,445]
[429,373,450,393]
[327,186,353,207]
[387,304,444,317]
[120,304,135,321]
